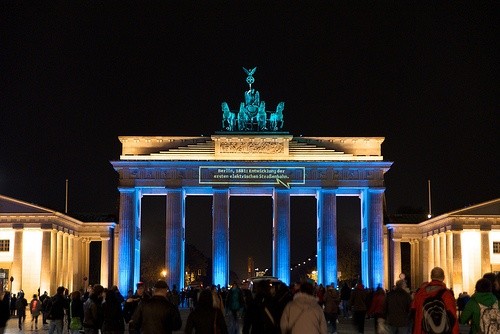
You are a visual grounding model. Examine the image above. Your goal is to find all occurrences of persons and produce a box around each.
[30,294,42,330]
[458,279,500,334]
[59,284,94,333]
[448,271,500,323]
[312,279,421,334]
[211,279,302,334]
[82,284,104,334]
[16,293,27,329]
[45,287,71,334]
[39,291,50,324]
[166,283,208,313]
[281,281,328,334]
[411,266,460,334]
[128,281,182,334]
[0,285,20,334]
[183,289,227,334]
[98,284,154,334]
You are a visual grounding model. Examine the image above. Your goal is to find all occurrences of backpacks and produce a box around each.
[472,294,500,334]
[422,288,452,334]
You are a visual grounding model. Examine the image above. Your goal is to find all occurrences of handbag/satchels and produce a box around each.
[70,317,82,330]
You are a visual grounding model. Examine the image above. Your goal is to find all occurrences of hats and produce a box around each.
[154,280,168,288]
